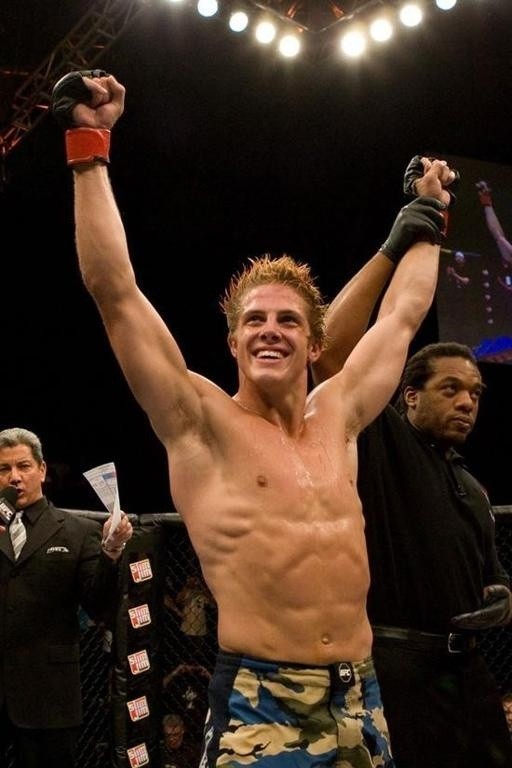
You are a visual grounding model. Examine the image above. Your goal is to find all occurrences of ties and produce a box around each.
[8,510,28,561]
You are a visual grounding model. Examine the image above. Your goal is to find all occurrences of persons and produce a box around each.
[445,252,474,291]
[78,571,215,768]
[504,694,512,730]
[456,180,511,363]
[307,191,512,767]
[44,62,462,767]
[1,427,130,768]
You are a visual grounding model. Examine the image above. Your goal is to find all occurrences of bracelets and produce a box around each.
[439,207,449,238]
[101,540,126,552]
[65,126,115,168]
[479,195,492,206]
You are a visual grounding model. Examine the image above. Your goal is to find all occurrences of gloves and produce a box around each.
[49,68,113,168]
[377,196,449,265]
[401,155,461,239]
[475,180,493,207]
[449,581,512,633]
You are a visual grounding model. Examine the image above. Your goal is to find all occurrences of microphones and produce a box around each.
[0,485,17,525]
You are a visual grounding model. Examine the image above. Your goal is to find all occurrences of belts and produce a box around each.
[371,624,477,656]
